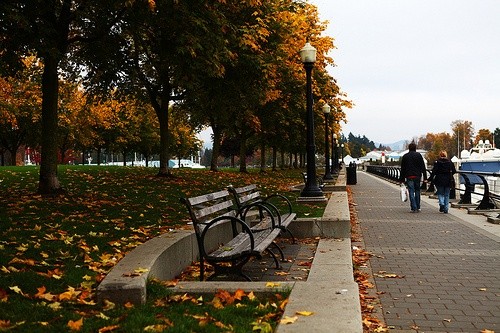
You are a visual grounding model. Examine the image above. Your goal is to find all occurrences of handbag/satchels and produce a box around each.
[400,183,408,202]
[450,178,454,188]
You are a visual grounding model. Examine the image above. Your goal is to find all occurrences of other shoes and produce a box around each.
[412,209,421,213]
[440,204,444,213]
[444,211,447,213]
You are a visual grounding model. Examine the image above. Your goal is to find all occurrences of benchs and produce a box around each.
[301,172,325,188]
[179,184,297,282]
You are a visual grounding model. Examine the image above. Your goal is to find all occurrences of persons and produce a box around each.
[349,162,353,167]
[399,144,427,213]
[425,150,457,214]
[353,161,356,171]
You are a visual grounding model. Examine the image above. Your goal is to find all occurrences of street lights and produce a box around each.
[320,102,336,185]
[340,143,345,164]
[330,106,341,177]
[296,36,329,204]
